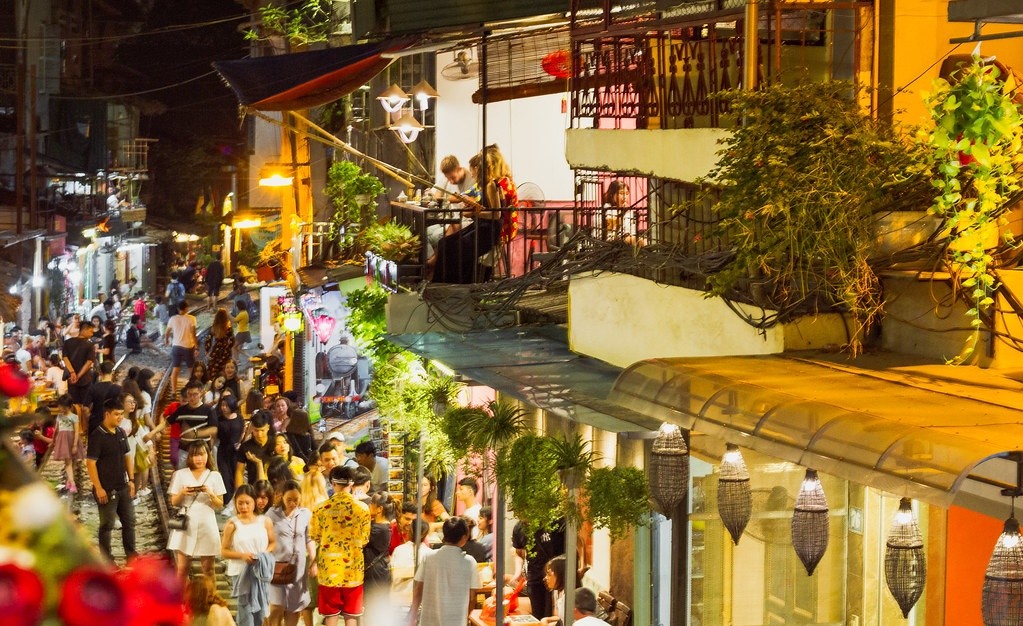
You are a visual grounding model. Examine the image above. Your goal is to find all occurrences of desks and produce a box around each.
[390,199,463,281]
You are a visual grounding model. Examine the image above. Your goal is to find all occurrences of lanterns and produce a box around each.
[543,49,585,114]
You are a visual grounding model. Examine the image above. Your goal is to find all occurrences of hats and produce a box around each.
[326,432,345,442]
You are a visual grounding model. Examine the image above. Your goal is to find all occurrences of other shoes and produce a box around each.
[131,495,141,506]
[70,485,77,493]
[65,481,71,490]
[138,487,151,496]
[237,361,242,366]
[170,394,177,400]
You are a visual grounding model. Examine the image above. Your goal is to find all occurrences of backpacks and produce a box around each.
[169,282,184,305]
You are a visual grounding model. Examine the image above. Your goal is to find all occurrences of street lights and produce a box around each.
[256,161,313,219]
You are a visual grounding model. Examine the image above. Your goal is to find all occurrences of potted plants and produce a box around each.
[460,398,534,455]
[337,171,391,247]
[505,433,609,561]
[344,283,471,431]
[581,466,655,546]
[321,159,363,242]
[424,406,474,483]
[926,56,1023,363]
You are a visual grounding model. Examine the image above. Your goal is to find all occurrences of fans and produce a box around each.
[496,182,546,282]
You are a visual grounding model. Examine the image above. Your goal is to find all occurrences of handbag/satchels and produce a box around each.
[62,367,71,381]
[167,506,189,530]
[270,561,297,584]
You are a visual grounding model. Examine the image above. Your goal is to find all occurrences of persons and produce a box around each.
[425,156,476,282]
[2,247,614,626]
[438,143,520,283]
[425,156,484,269]
[594,180,644,246]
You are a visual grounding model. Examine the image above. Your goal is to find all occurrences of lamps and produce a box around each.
[441,45,482,81]
[375,53,441,146]
[648,422,689,519]
[716,443,754,548]
[883,482,928,618]
[790,467,830,576]
[981,498,1023,625]
